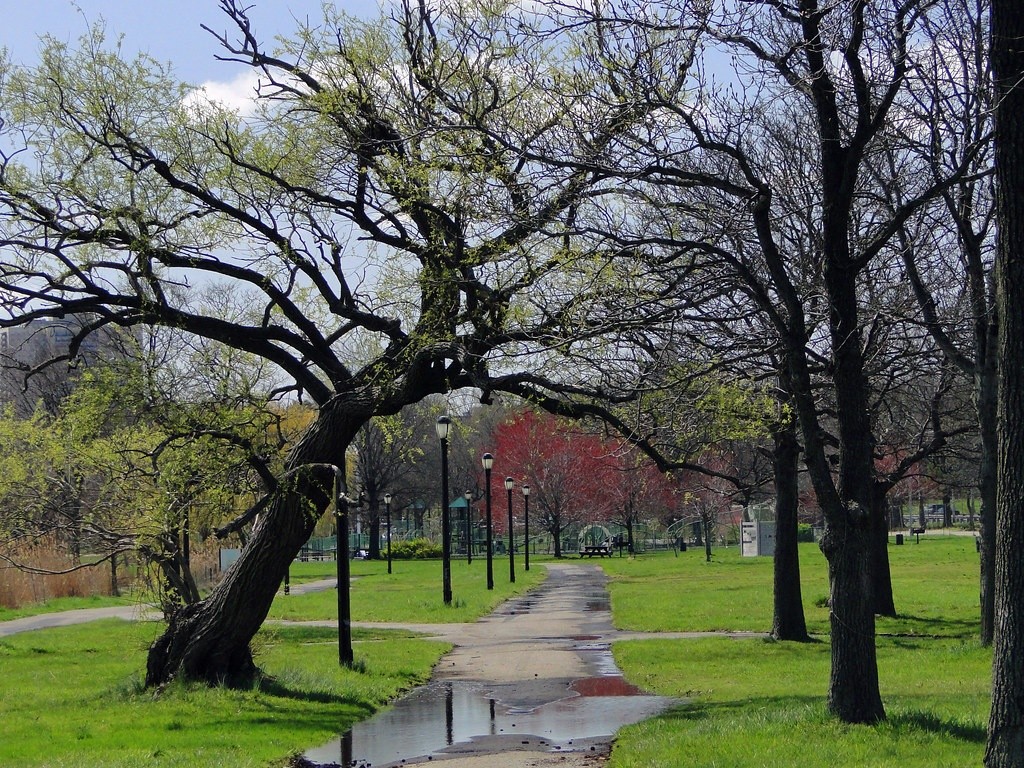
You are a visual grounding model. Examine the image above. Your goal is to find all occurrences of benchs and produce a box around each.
[578,546,612,559]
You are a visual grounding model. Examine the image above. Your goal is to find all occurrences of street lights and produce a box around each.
[384,493,393,574]
[481,451,495,589]
[435,411,458,595]
[522,483,531,570]
[503,476,517,583]
[466,489,475,564]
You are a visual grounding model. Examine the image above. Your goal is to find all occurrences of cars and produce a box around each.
[926,504,960,515]
